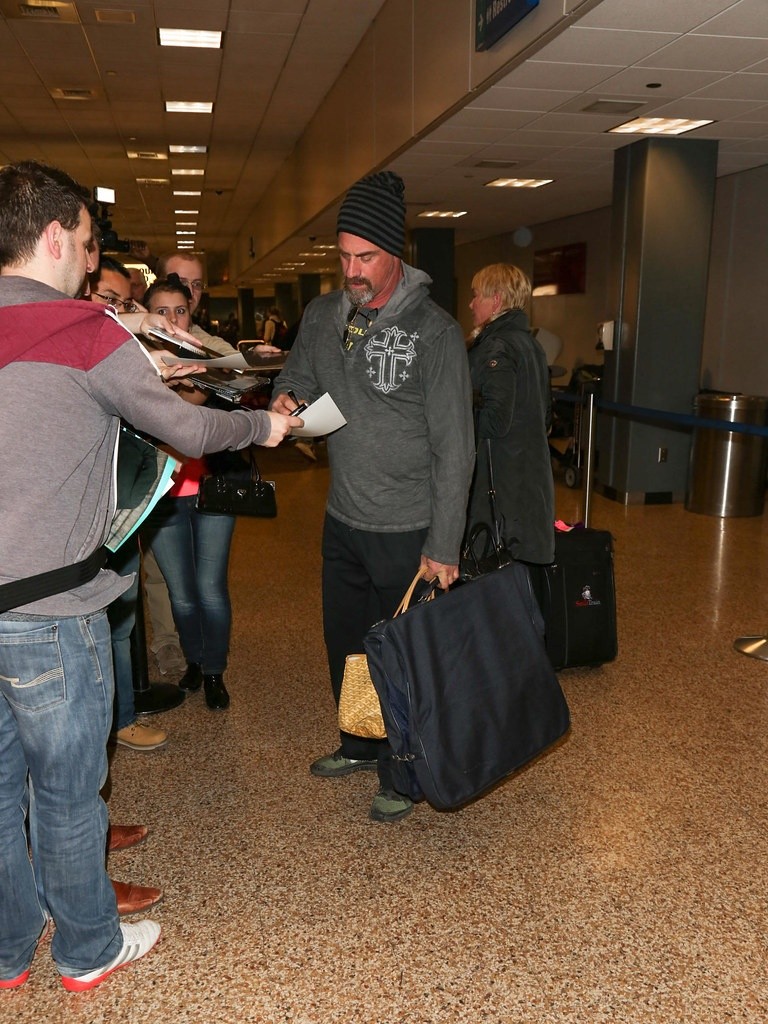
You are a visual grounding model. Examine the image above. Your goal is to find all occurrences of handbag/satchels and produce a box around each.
[338,653,386,739]
[196,445,278,517]
[460,522,515,580]
[365,561,572,812]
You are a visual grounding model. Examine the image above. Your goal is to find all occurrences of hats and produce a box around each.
[336,170,407,258]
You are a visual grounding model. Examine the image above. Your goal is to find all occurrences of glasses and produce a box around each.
[180,279,208,290]
[91,292,136,312]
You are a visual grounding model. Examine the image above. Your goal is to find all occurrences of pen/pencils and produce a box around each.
[288,387,308,420]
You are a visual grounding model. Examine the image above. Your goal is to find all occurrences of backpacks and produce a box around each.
[269,319,286,335]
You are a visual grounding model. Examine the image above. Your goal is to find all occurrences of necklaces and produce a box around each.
[344,304,360,347]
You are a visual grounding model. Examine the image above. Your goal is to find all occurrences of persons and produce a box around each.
[467,260,556,641]
[141,276,256,712]
[160,249,281,359]
[269,171,474,821]
[73,232,203,751]
[263,306,288,347]
[97,345,208,914]
[0,157,306,993]
[221,311,240,349]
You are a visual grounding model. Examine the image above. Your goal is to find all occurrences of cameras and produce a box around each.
[93,185,130,252]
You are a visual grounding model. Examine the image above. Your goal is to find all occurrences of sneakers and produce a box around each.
[310,748,378,776]
[0,909,49,988]
[110,720,168,750]
[61,919,161,991]
[369,789,414,822]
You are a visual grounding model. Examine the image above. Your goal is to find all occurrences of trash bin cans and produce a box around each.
[688,392,768,518]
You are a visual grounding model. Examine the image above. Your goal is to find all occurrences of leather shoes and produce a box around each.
[204,674,230,711]
[111,879,165,916]
[109,825,148,850]
[179,671,202,693]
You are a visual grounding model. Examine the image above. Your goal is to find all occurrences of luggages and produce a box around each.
[548,522,618,669]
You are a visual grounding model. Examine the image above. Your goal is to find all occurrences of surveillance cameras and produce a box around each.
[215,190,223,195]
